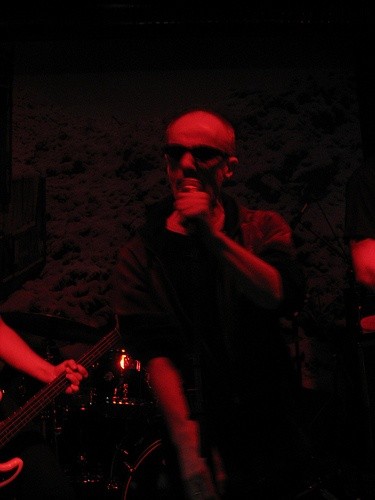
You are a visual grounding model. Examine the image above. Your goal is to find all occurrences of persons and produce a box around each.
[328,238,375,372]
[114,108,303,500]
[0,312,113,415]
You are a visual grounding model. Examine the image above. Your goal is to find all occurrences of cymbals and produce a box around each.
[1,310,99,342]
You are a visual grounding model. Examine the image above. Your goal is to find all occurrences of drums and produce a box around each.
[288,339,337,400]
[51,382,108,424]
[100,373,158,415]
[94,430,226,500]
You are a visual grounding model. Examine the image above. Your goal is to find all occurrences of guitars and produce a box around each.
[0,313,122,488]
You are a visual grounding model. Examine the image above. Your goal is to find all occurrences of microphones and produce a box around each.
[181,176,201,191]
[289,203,307,229]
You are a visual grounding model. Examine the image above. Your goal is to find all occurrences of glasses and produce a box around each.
[162,143,229,163]
